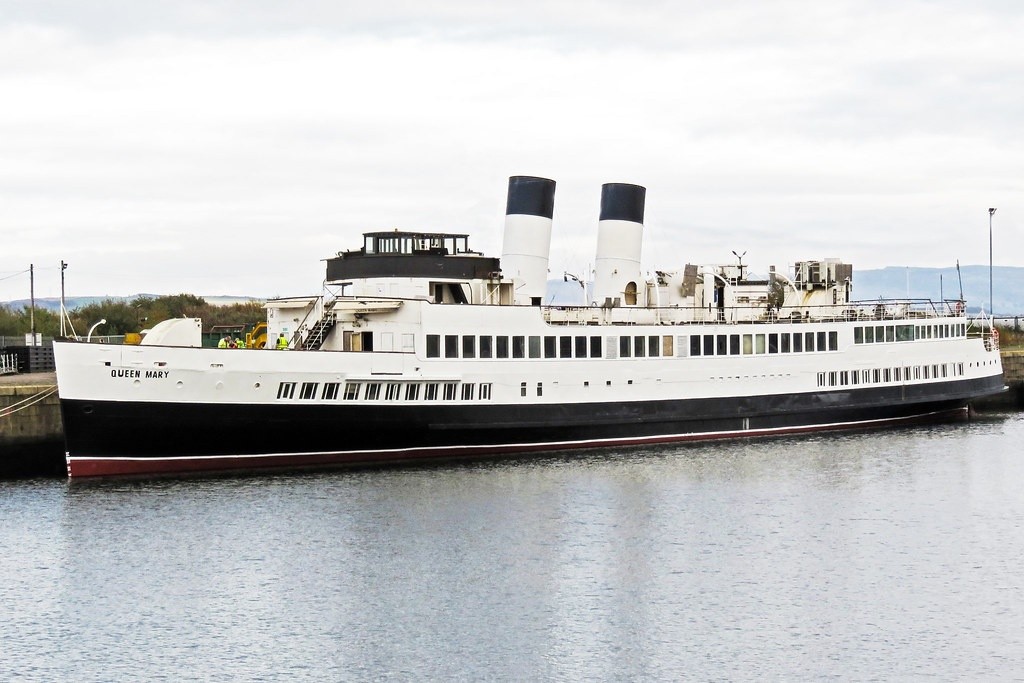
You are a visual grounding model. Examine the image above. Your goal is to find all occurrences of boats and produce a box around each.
[50,174,1011,479]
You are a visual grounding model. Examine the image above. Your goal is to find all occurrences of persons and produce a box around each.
[991,328,998,337]
[218,336,244,348]
[956,303,961,316]
[276,333,288,349]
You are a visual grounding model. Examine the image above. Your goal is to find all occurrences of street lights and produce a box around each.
[88,319,106,343]
[733,251,746,267]
[989,208,996,314]
[136,305,141,332]
